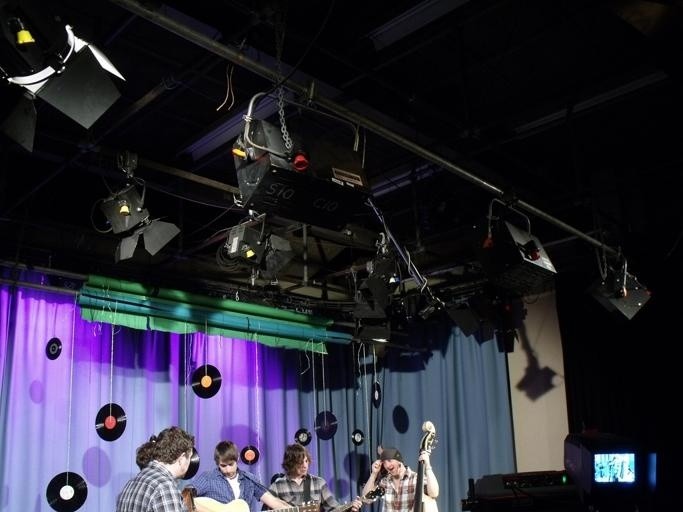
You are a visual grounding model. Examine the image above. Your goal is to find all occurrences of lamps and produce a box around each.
[360,318,391,344]
[418,302,437,320]
[469,198,557,297]
[589,247,651,321]
[100,183,180,265]
[355,274,388,320]
[2,10,127,153]
[225,224,267,267]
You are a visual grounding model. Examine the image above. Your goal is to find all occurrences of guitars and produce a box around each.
[192,496,318,512]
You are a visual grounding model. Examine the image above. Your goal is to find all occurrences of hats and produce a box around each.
[380,447,403,463]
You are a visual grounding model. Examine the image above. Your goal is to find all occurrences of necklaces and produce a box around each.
[229,471,237,480]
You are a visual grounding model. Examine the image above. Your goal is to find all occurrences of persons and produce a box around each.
[361,448,439,512]
[183,440,293,512]
[116,426,195,512]
[136,442,157,472]
[262,443,363,512]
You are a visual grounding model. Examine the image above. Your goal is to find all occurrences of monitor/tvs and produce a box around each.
[563,433,645,489]
